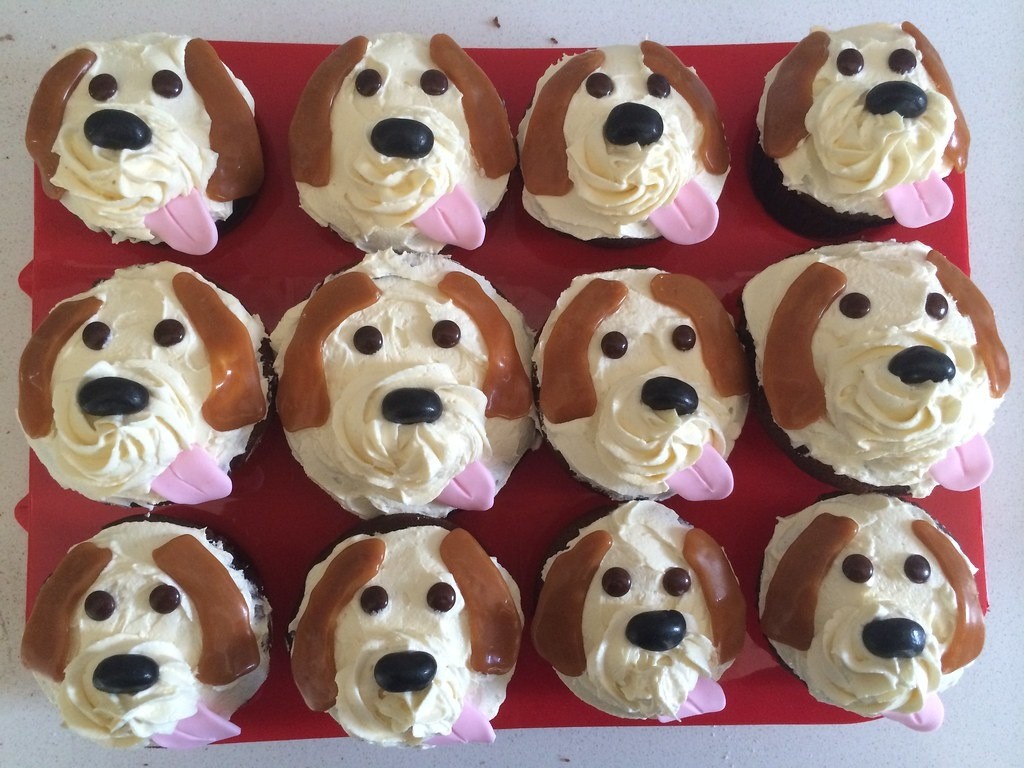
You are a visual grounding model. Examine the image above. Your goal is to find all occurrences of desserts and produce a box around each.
[25,20,1010,755]
